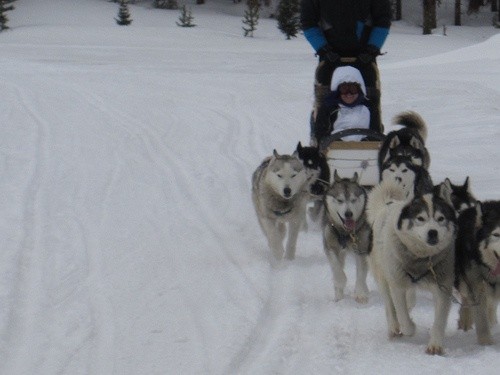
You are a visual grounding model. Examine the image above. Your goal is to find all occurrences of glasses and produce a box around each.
[337,83,361,95]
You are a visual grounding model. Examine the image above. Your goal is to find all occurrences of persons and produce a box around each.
[310,66,380,195]
[300,0,391,65]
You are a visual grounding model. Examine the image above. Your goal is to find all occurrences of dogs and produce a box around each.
[251,141,331,260]
[323,110,500,356]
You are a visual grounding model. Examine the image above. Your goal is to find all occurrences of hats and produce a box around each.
[329,65,370,101]
